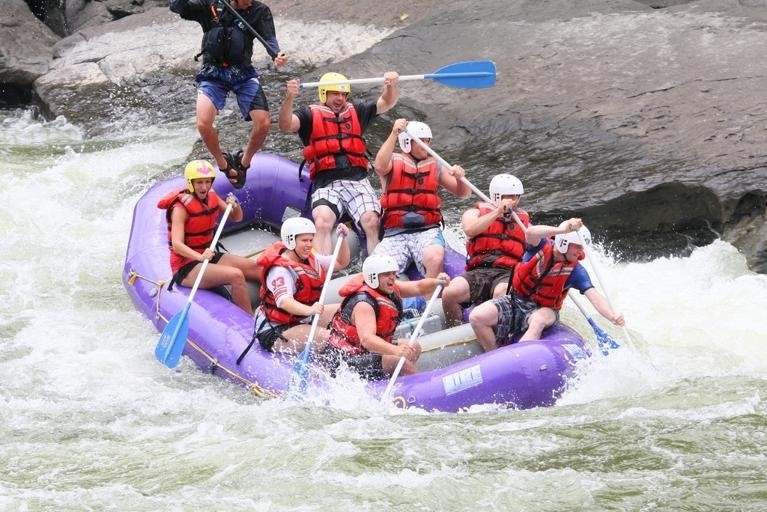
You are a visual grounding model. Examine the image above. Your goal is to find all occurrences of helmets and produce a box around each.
[281,217,317,250]
[555,219,592,253]
[185,160,216,193]
[489,173,524,207]
[319,72,351,103]
[362,254,399,289]
[398,121,433,153]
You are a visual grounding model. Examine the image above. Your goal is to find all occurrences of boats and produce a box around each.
[122,150,592,413]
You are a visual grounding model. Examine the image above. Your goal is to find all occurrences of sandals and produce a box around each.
[220,151,251,189]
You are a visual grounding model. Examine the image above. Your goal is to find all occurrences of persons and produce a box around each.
[279,71,400,262]
[443,172,534,327]
[170,0,288,189]
[253,216,351,354]
[159,157,265,316]
[467,216,625,352]
[318,253,450,381]
[369,117,472,300]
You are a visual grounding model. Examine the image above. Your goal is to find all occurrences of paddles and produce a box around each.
[300,61,496,88]
[510,208,620,356]
[155,193,237,369]
[288,232,344,396]
